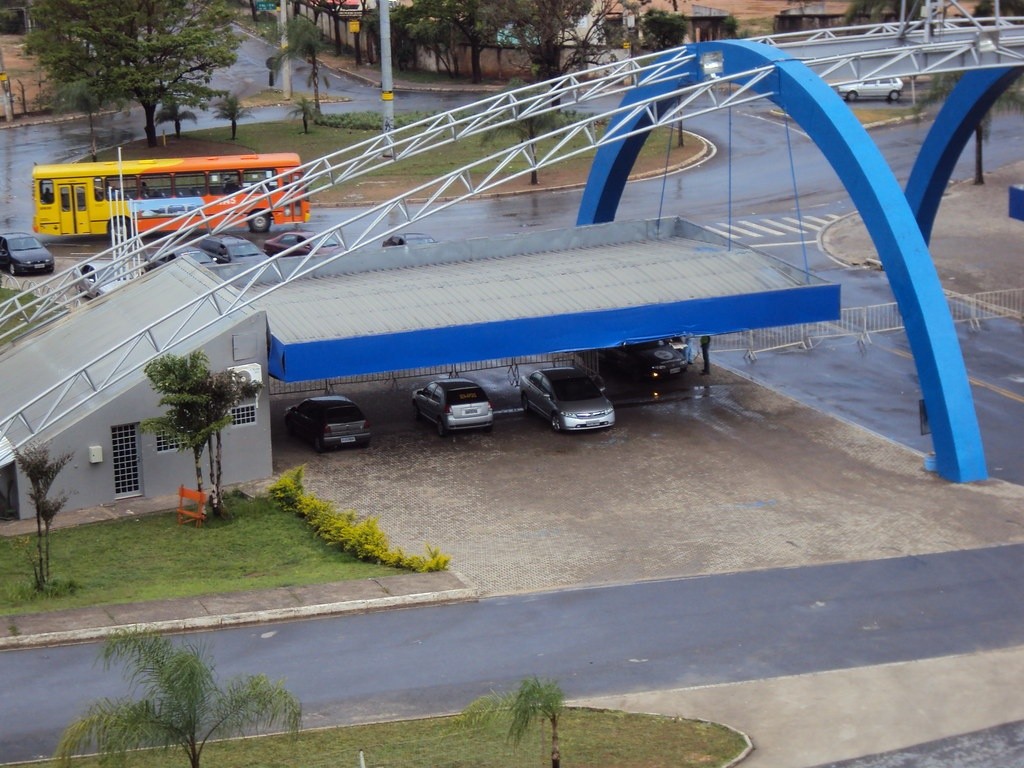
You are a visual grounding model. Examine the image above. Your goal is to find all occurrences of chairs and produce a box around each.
[11,239,22,249]
[23,238,37,248]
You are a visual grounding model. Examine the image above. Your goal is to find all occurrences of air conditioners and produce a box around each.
[226,363,263,392]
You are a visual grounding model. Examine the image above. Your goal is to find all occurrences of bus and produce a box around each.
[33,152,311,242]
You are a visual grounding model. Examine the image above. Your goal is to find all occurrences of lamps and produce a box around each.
[976,31,1000,53]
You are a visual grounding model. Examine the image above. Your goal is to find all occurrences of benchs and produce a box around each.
[44,188,54,204]
[176,190,192,197]
[194,187,204,196]
[155,190,167,197]
[143,192,153,199]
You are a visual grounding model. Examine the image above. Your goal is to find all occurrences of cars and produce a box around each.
[0,232,54,276]
[837,77,904,102]
[411,379,494,437]
[285,397,371,453]
[199,236,270,263]
[620,347,688,384]
[519,368,615,433]
[143,245,219,272]
[263,230,341,259]
[72,261,127,300]
[382,233,438,248]
